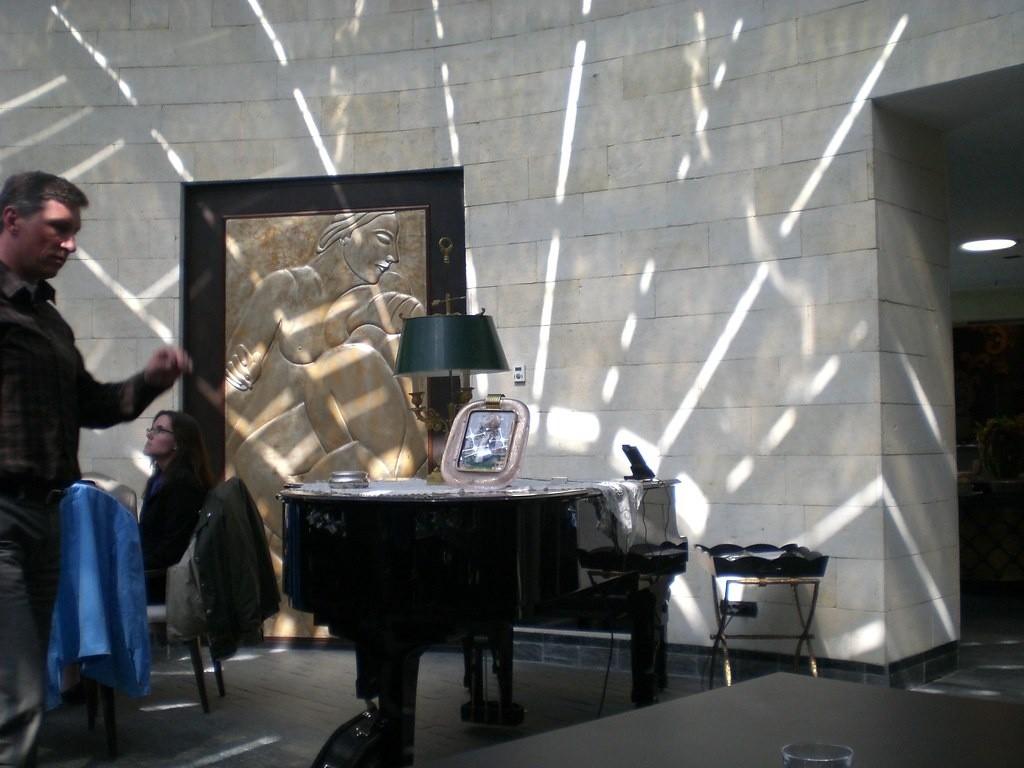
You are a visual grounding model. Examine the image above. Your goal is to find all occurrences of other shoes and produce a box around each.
[61,682,82,703]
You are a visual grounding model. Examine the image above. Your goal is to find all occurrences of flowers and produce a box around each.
[973,413,1024,480]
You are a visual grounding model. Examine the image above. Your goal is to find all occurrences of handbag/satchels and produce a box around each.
[311,707,387,768]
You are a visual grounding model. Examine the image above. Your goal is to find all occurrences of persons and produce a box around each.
[138,411,215,606]
[458,414,506,470]
[0,171,196,768]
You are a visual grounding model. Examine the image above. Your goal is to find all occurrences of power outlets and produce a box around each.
[719,600,758,618]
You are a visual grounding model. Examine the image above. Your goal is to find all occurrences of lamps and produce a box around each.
[392,236,511,486]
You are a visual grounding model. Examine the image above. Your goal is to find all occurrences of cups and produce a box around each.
[780,742,855,768]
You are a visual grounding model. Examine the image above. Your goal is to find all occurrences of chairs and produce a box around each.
[86,476,282,732]
[44,482,153,762]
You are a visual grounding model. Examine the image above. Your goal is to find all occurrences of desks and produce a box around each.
[416,669,1024,768]
[276,471,691,768]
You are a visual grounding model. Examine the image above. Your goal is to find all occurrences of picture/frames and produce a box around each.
[440,396,531,490]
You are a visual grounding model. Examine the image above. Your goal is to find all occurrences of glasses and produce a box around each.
[146,426,175,435]
